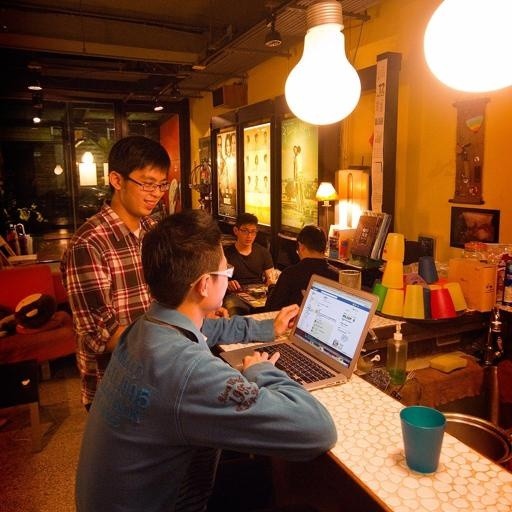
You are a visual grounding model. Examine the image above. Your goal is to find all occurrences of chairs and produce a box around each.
[1,264,77,379]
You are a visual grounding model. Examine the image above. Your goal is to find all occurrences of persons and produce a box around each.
[76,209,337,512]
[223,213,274,317]
[264,225,339,312]
[205,181,210,197]
[60,135,171,413]
[200,179,207,208]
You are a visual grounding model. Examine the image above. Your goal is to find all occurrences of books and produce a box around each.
[350,211,392,260]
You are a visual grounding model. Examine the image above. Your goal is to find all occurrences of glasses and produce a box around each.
[125,175,170,192]
[239,228,257,234]
[189,263,234,286]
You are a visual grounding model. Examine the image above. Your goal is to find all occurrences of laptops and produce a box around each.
[219,273,380,396]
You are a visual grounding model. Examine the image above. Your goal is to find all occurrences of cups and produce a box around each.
[372,232,469,322]
[399,406,447,474]
[338,269,362,292]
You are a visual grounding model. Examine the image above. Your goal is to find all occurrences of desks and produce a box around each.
[0,358,43,454]
[234,283,266,314]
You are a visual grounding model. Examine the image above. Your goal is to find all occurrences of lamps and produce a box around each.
[263,15,282,48]
